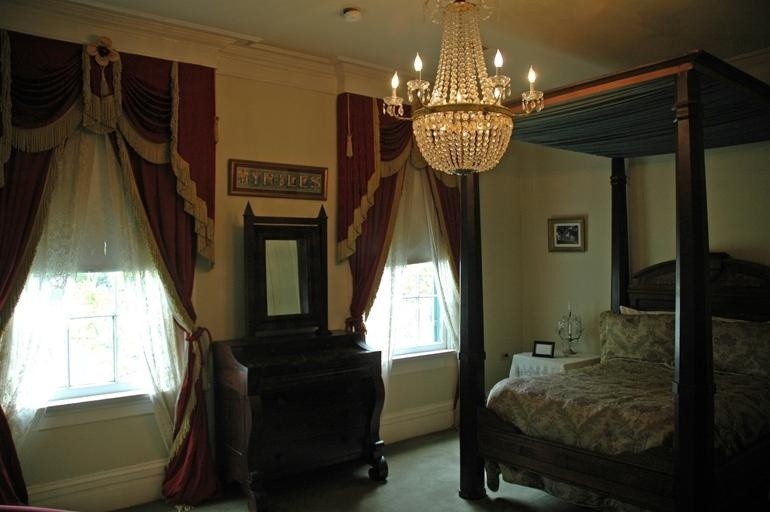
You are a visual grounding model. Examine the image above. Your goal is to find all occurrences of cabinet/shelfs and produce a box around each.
[212,330,388,512]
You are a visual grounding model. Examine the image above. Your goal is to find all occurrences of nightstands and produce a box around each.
[508,352,601,378]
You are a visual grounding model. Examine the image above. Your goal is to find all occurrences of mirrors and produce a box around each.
[241,199,330,341]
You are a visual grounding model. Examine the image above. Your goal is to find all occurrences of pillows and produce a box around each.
[599,305,770,377]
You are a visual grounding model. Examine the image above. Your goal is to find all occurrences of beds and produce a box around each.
[460,51,770,512]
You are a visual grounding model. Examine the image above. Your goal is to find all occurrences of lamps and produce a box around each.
[555,302,582,354]
[382,0,545,177]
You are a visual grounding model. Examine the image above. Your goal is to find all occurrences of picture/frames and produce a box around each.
[548,218,586,252]
[228,159,327,201]
[532,340,555,357]
